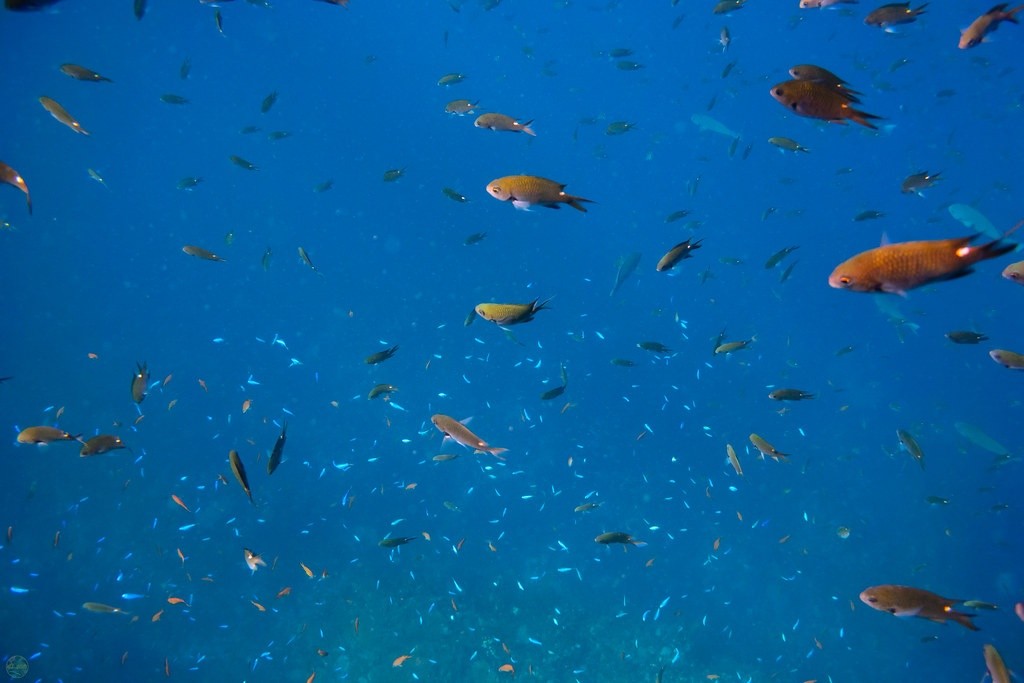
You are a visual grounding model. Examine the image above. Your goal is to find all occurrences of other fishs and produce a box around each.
[1,1,1024,677]
